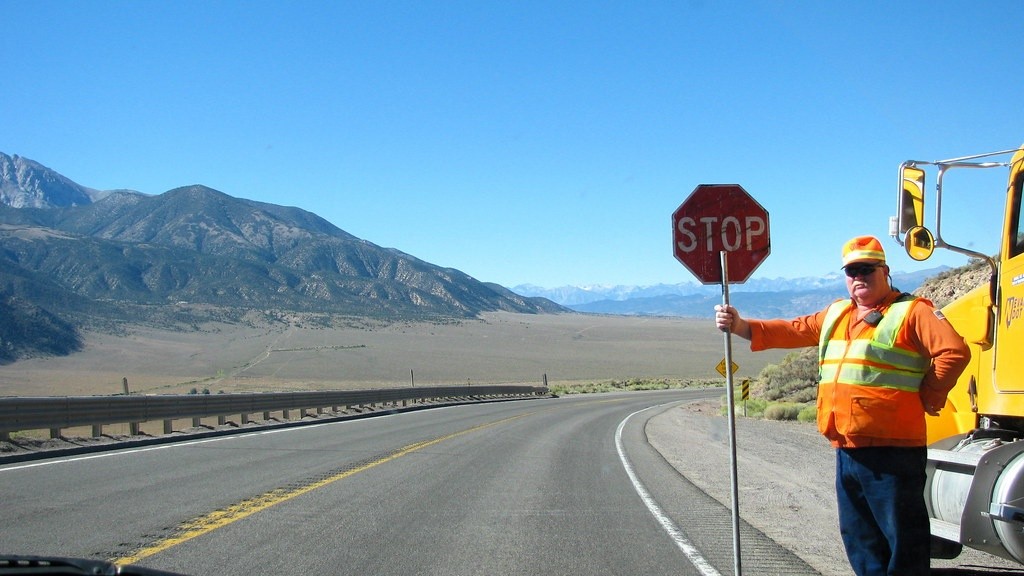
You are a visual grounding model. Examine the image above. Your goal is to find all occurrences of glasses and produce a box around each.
[845,265,884,277]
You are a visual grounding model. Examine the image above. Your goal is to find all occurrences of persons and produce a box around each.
[715,236,972,576]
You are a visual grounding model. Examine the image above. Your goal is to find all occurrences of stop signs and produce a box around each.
[670,184,773,285]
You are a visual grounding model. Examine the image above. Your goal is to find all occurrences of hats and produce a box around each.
[839,236,886,270]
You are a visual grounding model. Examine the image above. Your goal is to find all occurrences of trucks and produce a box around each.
[888,135,1024,567]
